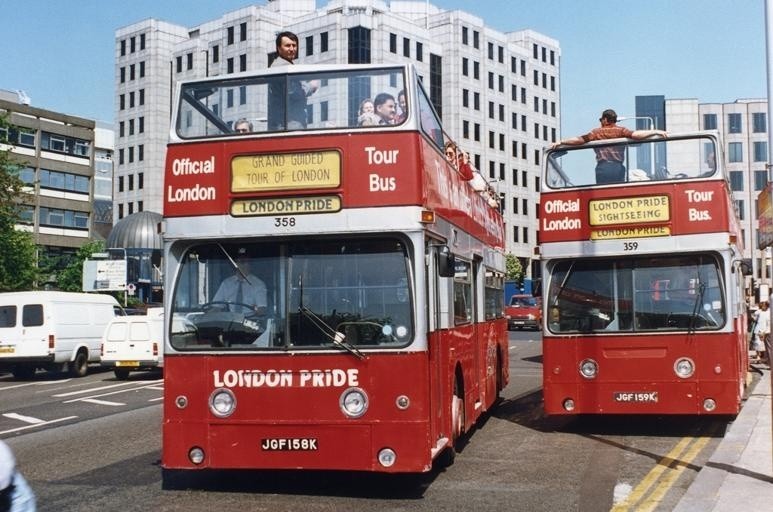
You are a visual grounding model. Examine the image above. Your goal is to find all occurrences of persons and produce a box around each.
[266,31,318,135]
[750,301,771,364]
[233,118,253,134]
[203,253,267,321]
[549,109,668,187]
[353,89,500,211]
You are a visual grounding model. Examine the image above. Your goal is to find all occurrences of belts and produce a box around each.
[597,160,623,164]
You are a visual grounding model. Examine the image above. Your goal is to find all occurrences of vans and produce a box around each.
[0,290,130,378]
[99,314,199,380]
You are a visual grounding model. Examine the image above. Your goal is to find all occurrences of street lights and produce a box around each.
[616,115,657,179]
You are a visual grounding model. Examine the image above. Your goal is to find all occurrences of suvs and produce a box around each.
[505,294,542,331]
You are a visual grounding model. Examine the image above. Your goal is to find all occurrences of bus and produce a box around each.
[158,60,511,477]
[537,128,750,418]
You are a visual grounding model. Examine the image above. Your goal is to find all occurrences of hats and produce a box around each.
[759,284,770,303]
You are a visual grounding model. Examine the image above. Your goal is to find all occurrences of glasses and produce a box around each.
[599,116,606,121]
[445,151,454,156]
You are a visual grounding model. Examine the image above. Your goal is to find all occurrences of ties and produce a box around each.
[235,282,243,315]
